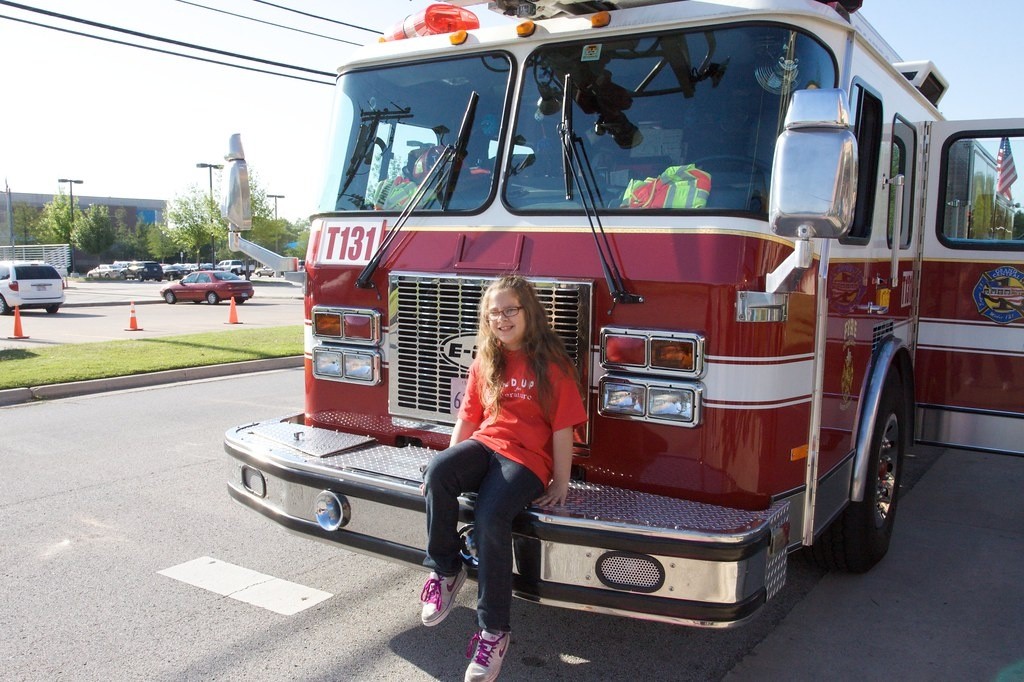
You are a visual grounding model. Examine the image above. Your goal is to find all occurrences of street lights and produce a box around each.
[266,195,285,254]
[196,163,224,270]
[58,178,83,273]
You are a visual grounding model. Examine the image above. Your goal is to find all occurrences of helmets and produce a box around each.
[411,147,464,185]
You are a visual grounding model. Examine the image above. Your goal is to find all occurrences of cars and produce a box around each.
[297,261,305,270]
[255,265,284,277]
[160,268,254,304]
[114,261,223,281]
[87,263,124,279]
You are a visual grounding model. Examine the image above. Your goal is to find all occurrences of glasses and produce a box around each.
[483,306,524,320]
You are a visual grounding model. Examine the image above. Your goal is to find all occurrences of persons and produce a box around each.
[418,276,588,682]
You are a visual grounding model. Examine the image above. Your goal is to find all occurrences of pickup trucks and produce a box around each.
[220,260,256,275]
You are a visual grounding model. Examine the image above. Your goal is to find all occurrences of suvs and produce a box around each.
[0,261,65,314]
[120,262,163,282]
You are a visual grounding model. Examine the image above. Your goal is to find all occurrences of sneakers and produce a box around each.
[420,562,467,627]
[464,629,510,682]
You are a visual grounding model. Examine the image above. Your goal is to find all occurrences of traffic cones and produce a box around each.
[125,302,144,332]
[8,304,30,340]
[223,296,242,324]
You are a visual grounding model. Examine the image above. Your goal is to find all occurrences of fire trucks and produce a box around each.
[225,0,1024,629]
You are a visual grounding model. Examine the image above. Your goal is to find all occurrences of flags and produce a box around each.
[996,137,1018,201]
